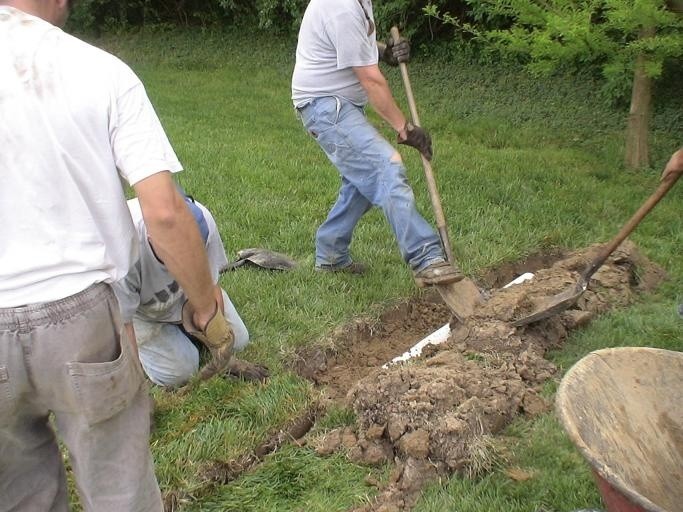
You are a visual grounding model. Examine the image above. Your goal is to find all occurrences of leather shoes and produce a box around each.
[341,262,369,274]
[413,262,465,288]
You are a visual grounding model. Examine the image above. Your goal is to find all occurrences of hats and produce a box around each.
[177,185,209,248]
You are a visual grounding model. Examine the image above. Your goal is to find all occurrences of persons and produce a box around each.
[114,188,272,435]
[0,1,231,511]
[660,148,682,178]
[290,0,464,288]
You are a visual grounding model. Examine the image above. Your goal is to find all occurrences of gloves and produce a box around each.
[397,119,432,161]
[383,37,413,67]
[181,298,235,381]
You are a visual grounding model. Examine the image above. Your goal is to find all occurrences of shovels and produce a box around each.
[219,248,297,274]
[391,27,484,325]
[513,171,681,326]
